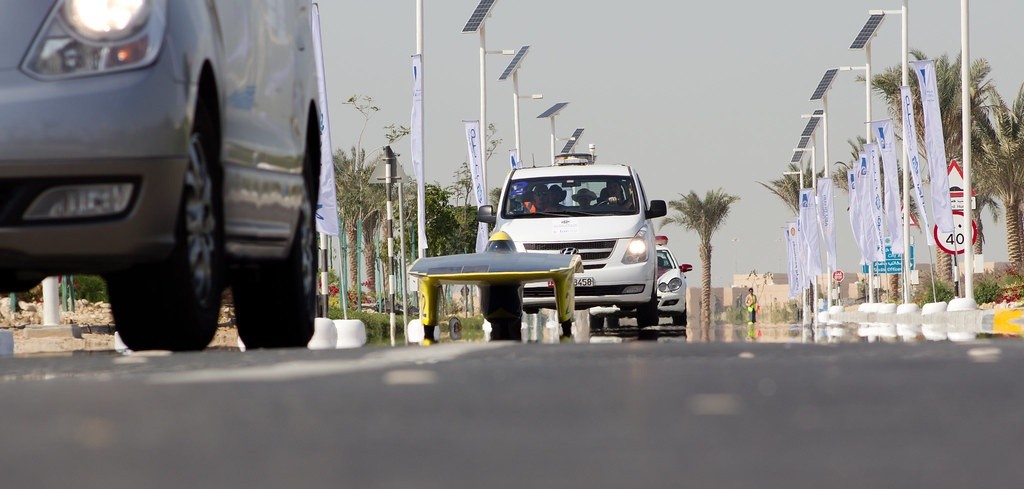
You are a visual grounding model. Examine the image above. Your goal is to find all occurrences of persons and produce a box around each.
[745,288,758,326]
[509,180,631,211]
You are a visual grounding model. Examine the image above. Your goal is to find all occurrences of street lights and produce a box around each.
[781,0,913,307]
[460,0,516,246]
[497,45,543,168]
[536,102,584,167]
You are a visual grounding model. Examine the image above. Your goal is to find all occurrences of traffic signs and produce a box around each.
[861,235,915,274]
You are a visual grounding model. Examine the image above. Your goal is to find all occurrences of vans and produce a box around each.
[0,0,331,354]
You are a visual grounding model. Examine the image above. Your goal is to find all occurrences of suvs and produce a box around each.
[588,246,694,331]
[475,161,668,342]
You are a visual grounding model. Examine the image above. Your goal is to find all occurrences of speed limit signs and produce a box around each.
[933,211,978,255]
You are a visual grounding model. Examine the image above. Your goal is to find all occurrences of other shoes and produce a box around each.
[748,322,753,325]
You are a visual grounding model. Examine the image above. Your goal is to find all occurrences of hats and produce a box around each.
[548,185,567,202]
[572,187,596,202]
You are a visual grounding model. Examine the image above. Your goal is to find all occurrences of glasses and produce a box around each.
[536,191,547,196]
